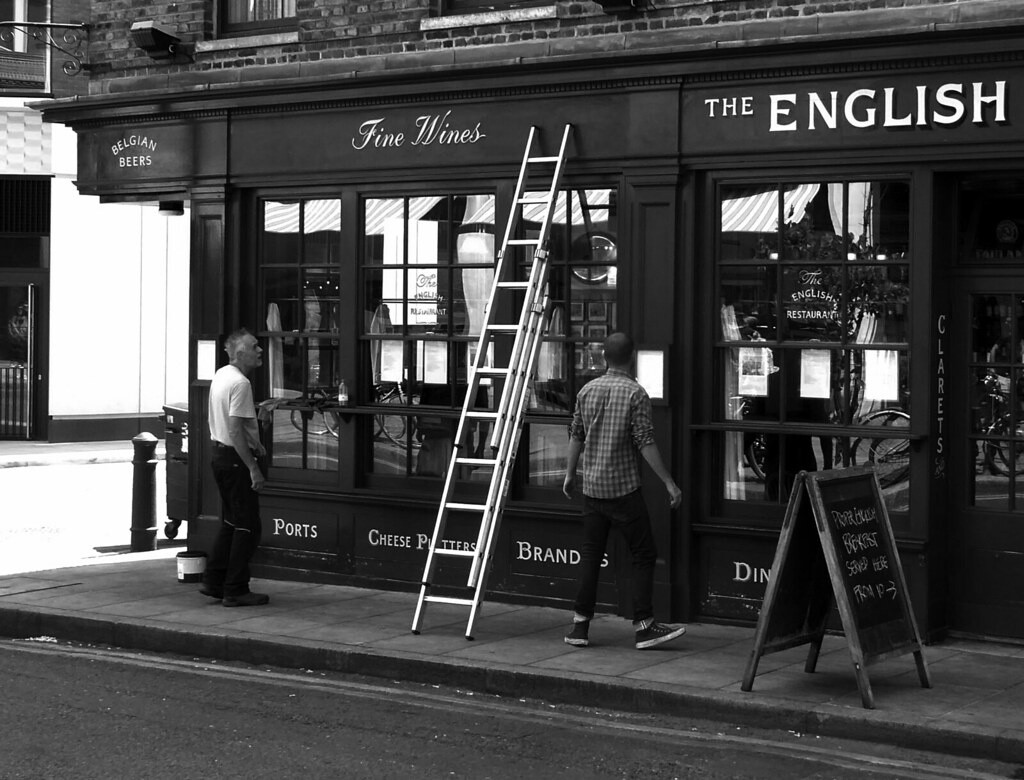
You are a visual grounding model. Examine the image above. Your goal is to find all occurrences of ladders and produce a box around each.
[411,120,572,639]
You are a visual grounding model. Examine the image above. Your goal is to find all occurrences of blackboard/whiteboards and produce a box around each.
[811,460,923,668]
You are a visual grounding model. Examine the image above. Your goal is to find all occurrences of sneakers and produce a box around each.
[198,579,225,599]
[223,591,270,607]
[634,617,685,648]
[564,632,588,645]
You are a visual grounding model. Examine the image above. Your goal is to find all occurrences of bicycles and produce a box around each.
[742,359,1024,491]
[290,365,427,450]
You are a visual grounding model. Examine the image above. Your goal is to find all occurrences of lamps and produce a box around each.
[130,20,179,59]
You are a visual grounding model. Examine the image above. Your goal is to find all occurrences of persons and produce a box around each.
[562,331,685,648]
[199,328,269,606]
